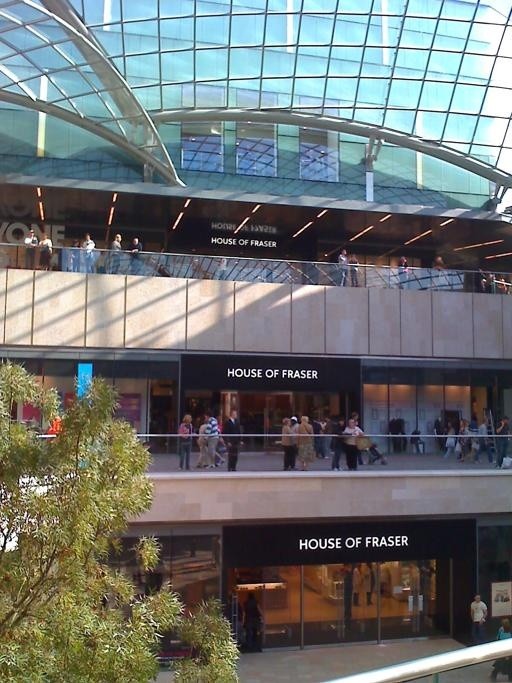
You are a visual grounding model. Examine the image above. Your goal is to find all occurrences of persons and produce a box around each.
[488,617,512,682]
[69,239,82,272]
[337,248,359,287]
[128,237,143,274]
[217,258,227,280]
[243,591,264,653]
[478,266,510,294]
[24,229,37,269]
[279,411,512,470]
[176,408,245,471]
[470,594,488,646]
[364,562,376,607]
[111,233,122,274]
[39,233,53,270]
[82,233,96,274]
[351,562,362,608]
[431,255,448,269]
[380,562,391,596]
[398,256,409,289]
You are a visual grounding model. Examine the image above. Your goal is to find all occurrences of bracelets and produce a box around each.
[482,618,486,622]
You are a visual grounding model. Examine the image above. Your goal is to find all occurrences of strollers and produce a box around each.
[365,446,387,464]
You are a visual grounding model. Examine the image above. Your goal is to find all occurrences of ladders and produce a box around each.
[483,408,498,454]
